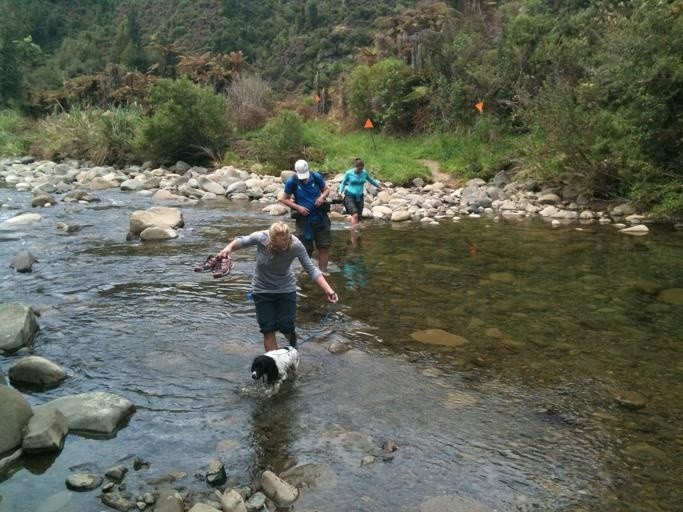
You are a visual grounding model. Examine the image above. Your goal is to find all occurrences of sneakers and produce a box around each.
[213,257,232,278]
[194,254,220,273]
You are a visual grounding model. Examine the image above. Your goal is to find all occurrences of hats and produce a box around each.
[295,160,310,180]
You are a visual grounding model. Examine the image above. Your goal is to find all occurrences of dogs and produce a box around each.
[250,344,301,398]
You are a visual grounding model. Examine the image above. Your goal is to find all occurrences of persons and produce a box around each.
[281,159,333,281]
[215,221,339,351]
[338,158,385,225]
